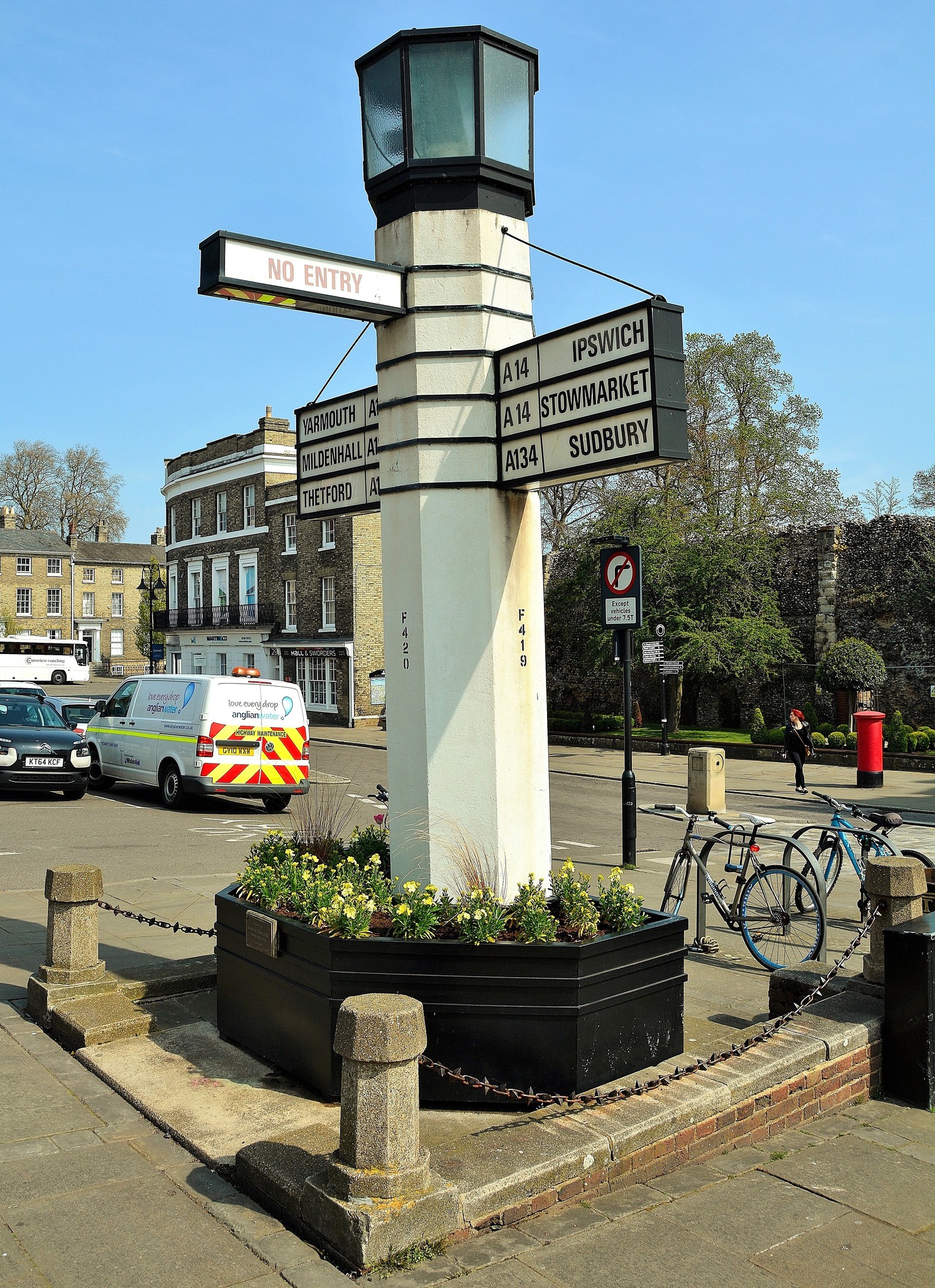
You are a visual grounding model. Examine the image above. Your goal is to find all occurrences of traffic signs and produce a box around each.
[642,641,664,664]
[659,660,683,674]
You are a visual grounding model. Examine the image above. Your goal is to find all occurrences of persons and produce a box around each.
[783,710,815,793]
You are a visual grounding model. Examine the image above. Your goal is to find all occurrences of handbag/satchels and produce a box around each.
[805,745,811,756]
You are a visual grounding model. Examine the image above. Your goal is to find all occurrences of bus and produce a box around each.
[0,634,90,685]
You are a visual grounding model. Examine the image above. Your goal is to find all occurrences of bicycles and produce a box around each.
[340,784,389,876]
[656,804,825,973]
[795,791,935,913]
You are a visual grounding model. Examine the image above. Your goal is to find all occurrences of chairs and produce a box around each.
[5,710,29,727]
[2,648,61,655]
[79,709,94,723]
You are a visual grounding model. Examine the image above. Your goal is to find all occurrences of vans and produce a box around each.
[85,667,310,812]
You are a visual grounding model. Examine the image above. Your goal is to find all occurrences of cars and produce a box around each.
[0,682,120,800]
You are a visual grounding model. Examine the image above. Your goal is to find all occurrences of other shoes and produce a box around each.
[803,790,808,794]
[795,787,804,793]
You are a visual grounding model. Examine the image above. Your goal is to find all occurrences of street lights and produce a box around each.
[135,564,167,676]
